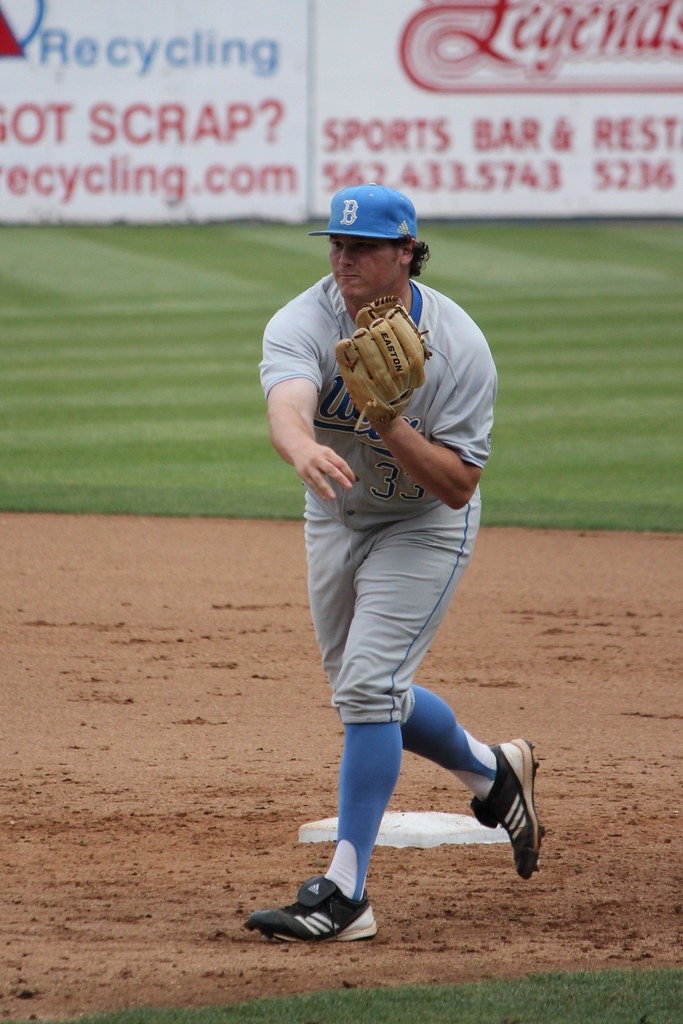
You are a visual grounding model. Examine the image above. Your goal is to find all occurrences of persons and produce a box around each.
[245,182,544,942]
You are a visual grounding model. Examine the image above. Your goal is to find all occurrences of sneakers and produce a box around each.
[244,875,378,944]
[470,738,544,881]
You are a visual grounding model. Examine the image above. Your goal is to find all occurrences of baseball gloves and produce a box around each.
[333,294,432,434]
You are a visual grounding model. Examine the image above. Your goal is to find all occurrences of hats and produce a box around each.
[307,183,417,240]
